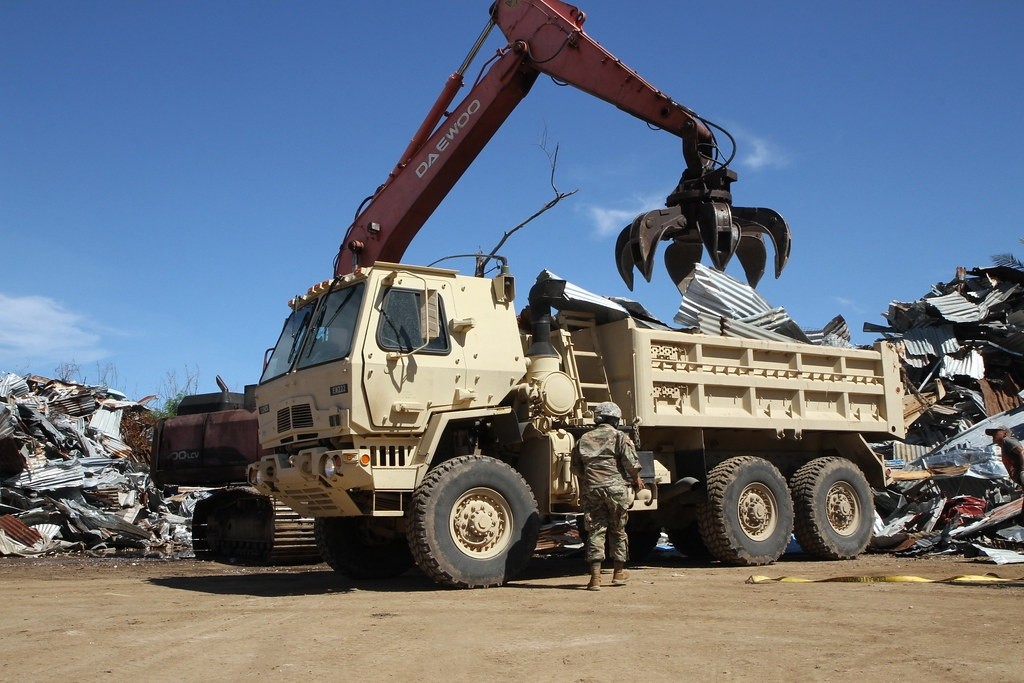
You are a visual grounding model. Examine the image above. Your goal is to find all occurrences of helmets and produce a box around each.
[593,402,621,423]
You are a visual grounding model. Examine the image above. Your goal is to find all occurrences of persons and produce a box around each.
[571,401,645,591]
[985,425,1024,554]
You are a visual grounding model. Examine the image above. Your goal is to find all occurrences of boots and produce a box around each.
[587,563,603,592]
[612,563,630,585]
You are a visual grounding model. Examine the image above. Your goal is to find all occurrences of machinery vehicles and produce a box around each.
[148,1,790,566]
[250,259,904,589]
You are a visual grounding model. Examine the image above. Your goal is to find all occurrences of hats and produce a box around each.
[985,425,1010,437]
